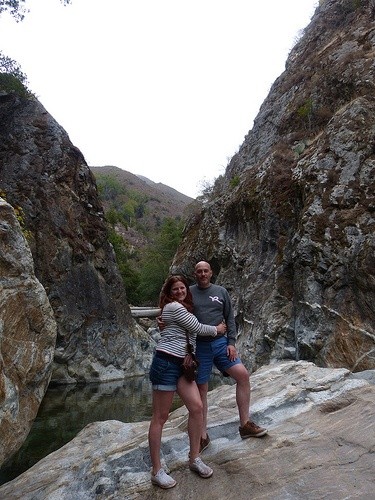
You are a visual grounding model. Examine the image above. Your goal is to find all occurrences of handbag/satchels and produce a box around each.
[182,353,200,380]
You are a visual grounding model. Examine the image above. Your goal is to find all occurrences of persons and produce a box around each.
[148,275,227,489]
[155,261,268,459]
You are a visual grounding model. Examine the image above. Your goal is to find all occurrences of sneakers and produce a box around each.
[151,468,177,488]
[188,433,210,458]
[189,457,213,477]
[239,421,268,438]
